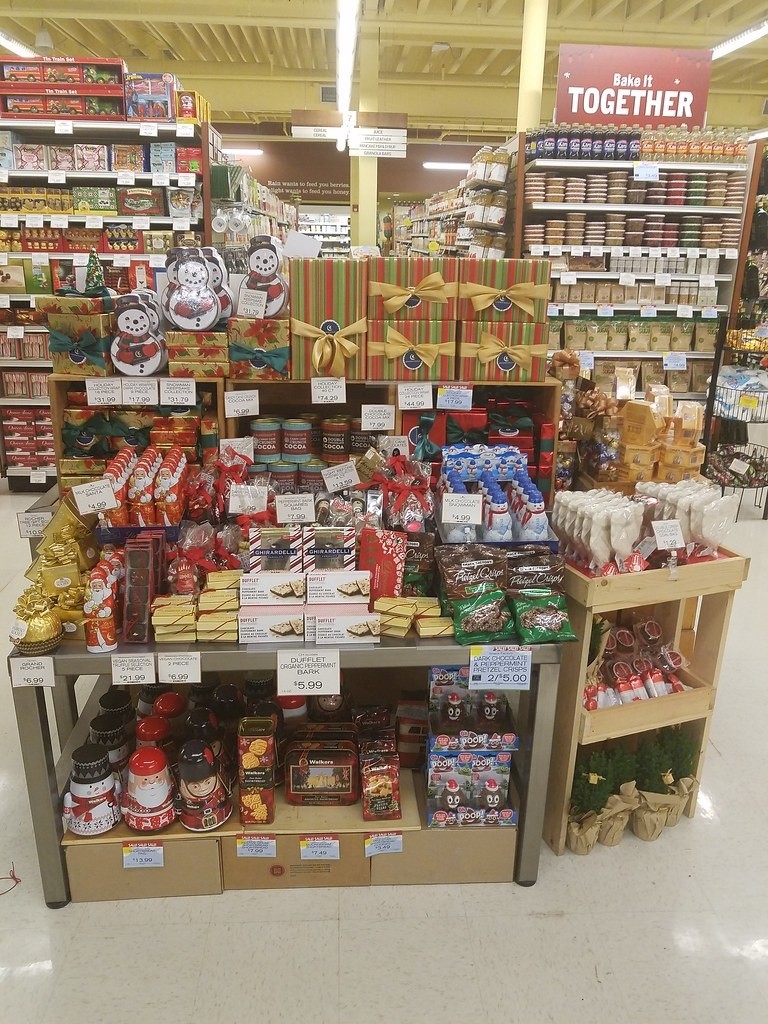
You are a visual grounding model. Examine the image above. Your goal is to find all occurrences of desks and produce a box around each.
[6,643,564,909]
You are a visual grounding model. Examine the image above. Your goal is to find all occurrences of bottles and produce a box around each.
[428,146,510,258]
[524,122,751,164]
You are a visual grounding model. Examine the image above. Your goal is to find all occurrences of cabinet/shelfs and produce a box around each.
[0,121,213,494]
[505,130,768,494]
[298,212,351,257]
[543,545,750,855]
[394,145,518,258]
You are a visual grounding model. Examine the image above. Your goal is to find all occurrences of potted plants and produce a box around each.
[631,739,669,841]
[599,742,639,846]
[659,728,699,827]
[565,750,606,856]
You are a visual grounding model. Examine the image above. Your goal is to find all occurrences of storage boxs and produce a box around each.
[0,55,126,122]
[401,389,555,511]
[237,717,276,825]
[238,610,304,643]
[368,257,458,321]
[371,829,517,888]
[458,257,550,323]
[220,832,370,890]
[368,321,455,381]
[459,321,550,383]
[64,840,223,903]
[96,508,187,550]
[0,297,289,472]
[315,612,380,644]
[239,527,371,609]
[24,491,240,643]
[58,389,218,497]
[287,256,367,381]
[359,528,407,612]
[433,516,559,554]
[123,71,212,123]
